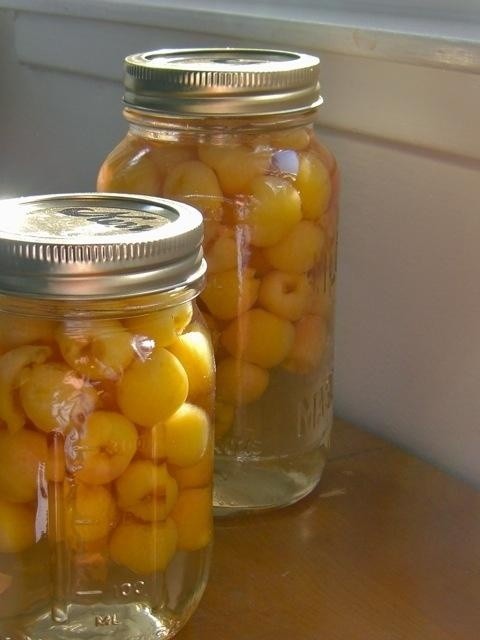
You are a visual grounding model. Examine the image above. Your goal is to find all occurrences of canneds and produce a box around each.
[0,191,220,640]
[95,44,341,519]
